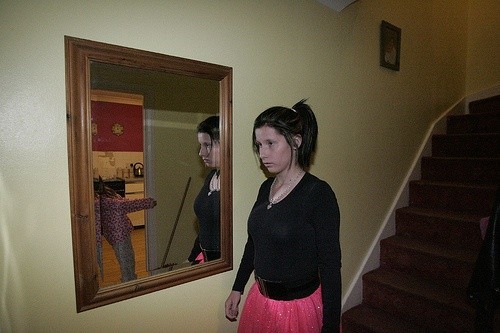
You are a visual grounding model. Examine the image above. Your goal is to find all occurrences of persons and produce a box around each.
[223,99,342,333]
[183,116,222,266]
[94,176,158,283]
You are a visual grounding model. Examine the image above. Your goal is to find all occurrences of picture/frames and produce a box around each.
[379,21,401,71]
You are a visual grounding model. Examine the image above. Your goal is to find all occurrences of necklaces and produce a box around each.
[207,173,220,196]
[266,168,304,209]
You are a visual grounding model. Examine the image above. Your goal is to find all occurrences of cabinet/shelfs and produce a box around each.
[124,183,145,227]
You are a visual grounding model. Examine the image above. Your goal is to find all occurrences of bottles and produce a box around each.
[108,160,130,178]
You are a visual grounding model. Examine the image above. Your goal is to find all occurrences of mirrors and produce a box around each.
[63,36,234,314]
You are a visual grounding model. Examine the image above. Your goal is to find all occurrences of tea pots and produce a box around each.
[130,161,144,177]
